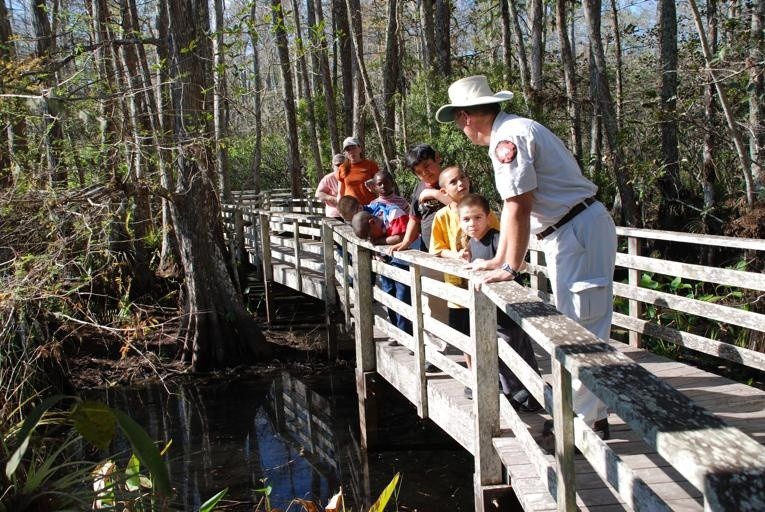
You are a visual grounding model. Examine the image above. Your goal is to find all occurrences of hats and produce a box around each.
[343,137,360,151]
[333,154,345,164]
[436,75,514,123]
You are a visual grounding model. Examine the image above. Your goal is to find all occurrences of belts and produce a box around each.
[536,196,596,241]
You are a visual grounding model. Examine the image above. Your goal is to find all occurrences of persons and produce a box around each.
[435,76,618,455]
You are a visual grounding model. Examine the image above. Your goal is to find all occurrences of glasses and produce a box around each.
[334,164,340,167]
